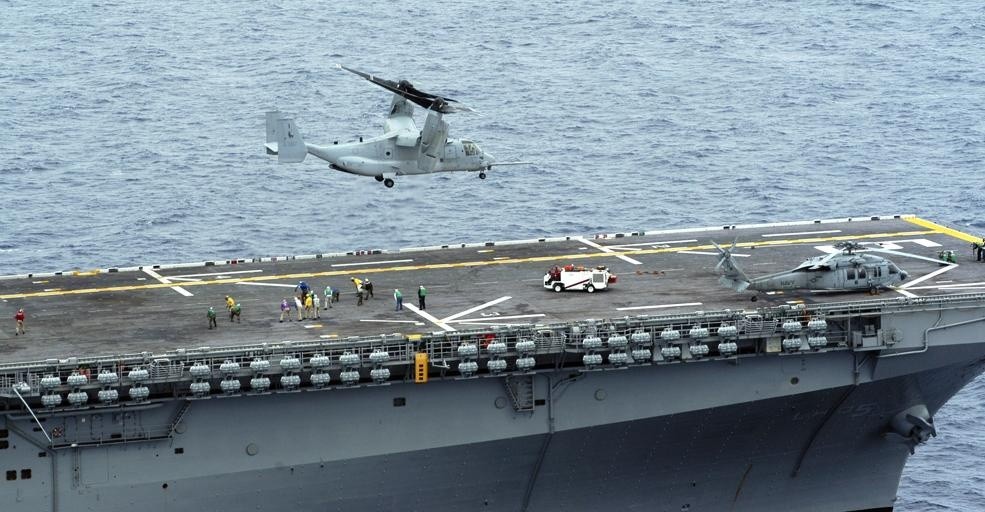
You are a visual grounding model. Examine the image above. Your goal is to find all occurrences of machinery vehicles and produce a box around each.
[543,265,617,294]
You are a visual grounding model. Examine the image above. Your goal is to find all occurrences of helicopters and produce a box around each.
[263,65,534,189]
[709,233,925,303]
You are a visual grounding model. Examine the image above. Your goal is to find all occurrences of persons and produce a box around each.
[545,266,559,288]
[207,306,217,328]
[224,276,373,323]
[418,282,427,310]
[932,236,985,267]
[394,289,404,310]
[12,307,28,337]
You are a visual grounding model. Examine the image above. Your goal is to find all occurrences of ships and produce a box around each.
[0,213,985,511]
